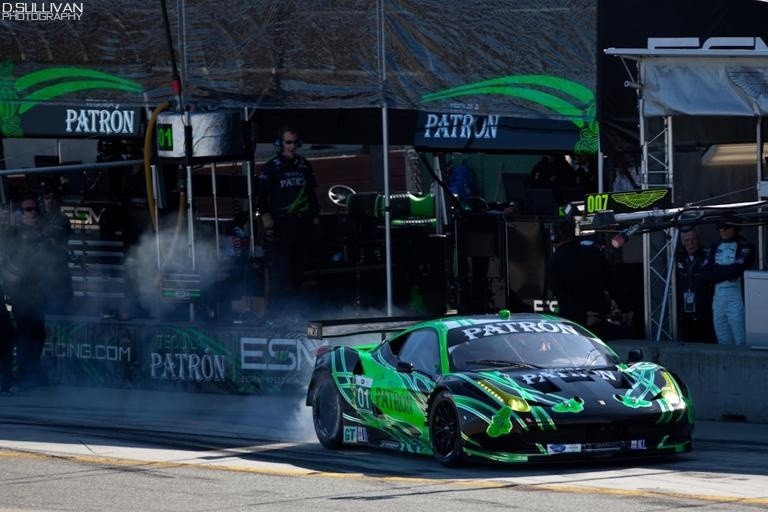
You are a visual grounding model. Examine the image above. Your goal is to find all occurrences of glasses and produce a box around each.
[22,206,40,212]
[285,140,298,145]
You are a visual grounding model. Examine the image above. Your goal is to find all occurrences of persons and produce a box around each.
[40,192,76,314]
[1,198,62,399]
[705,224,752,345]
[674,225,710,342]
[252,122,326,332]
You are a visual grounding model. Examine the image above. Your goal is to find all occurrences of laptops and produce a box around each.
[527,188,555,215]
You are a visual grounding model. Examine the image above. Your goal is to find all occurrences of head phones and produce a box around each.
[14,206,24,217]
[272,137,303,148]
[716,221,744,232]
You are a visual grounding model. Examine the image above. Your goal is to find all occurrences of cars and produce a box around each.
[309,309,694,469]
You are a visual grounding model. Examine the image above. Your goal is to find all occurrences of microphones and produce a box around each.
[611,223,641,249]
